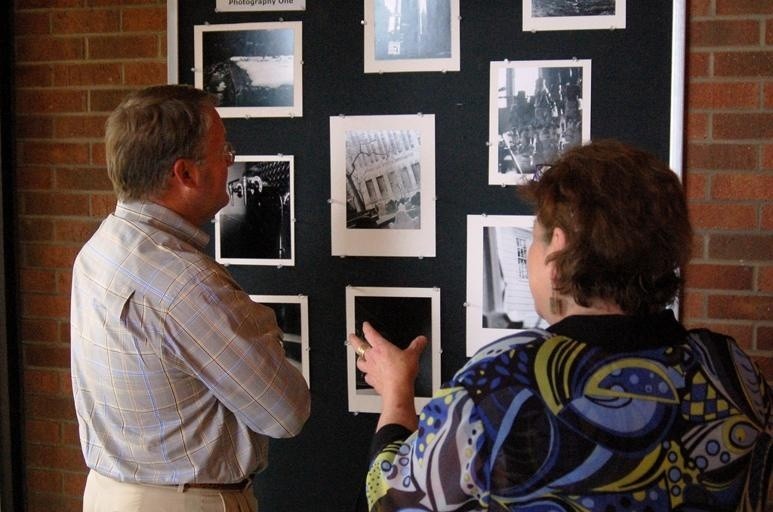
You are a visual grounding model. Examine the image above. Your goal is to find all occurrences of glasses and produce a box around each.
[218,140,236,165]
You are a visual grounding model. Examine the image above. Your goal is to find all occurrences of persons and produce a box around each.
[347,140,773,511]
[67,84,313,512]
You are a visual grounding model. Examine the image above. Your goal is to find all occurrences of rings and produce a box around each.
[356,342,371,362]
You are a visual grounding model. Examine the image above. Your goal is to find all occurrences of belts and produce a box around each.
[171,473,255,491]
